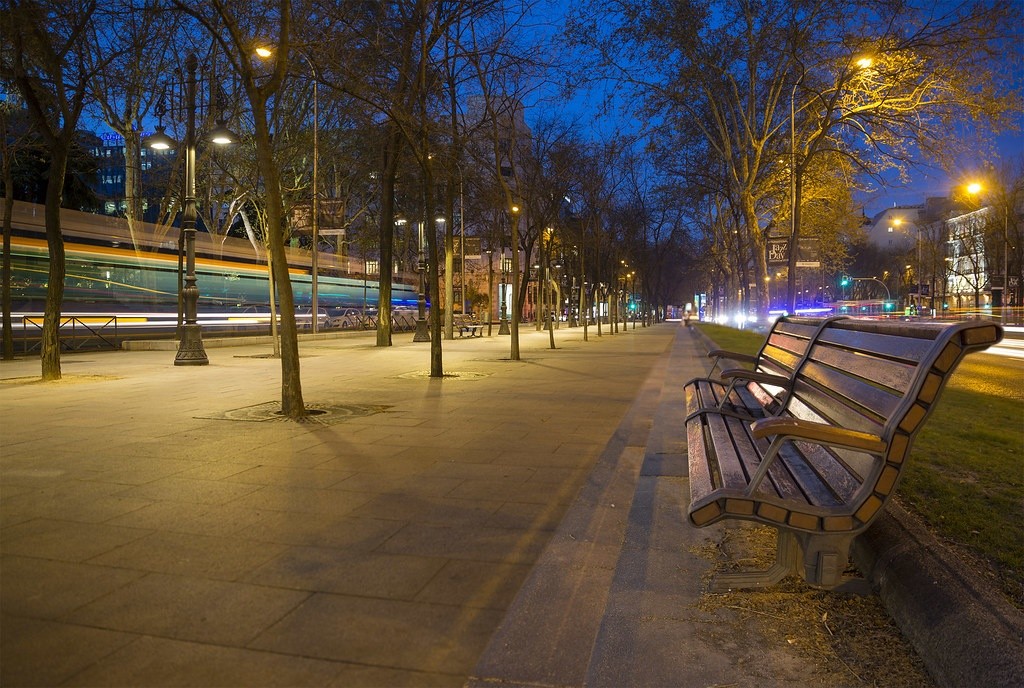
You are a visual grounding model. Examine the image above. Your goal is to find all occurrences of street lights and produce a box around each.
[892,217,921,310]
[968,182,1007,328]
[255,41,320,336]
[144,51,239,367]
[427,151,466,315]
[790,52,875,235]
[482,242,496,337]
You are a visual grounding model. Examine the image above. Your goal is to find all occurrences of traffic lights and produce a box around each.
[842,276,848,286]
[630,303,636,309]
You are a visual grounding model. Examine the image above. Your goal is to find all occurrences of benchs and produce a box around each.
[453,313,484,338]
[682,311,1005,594]
